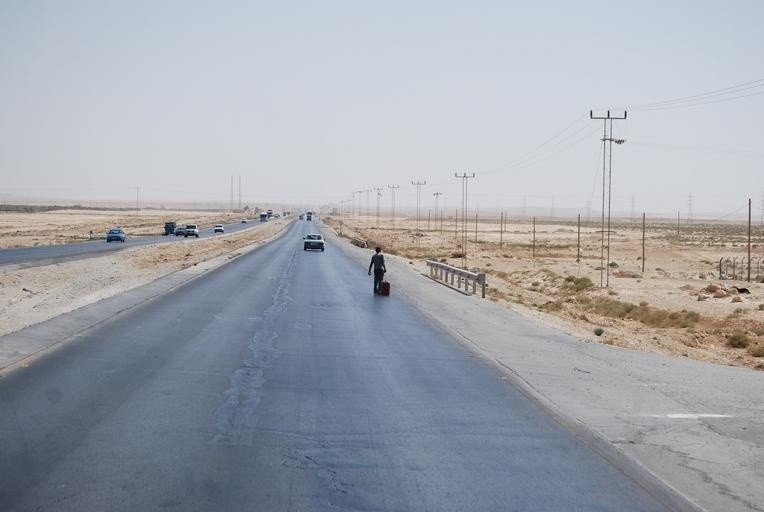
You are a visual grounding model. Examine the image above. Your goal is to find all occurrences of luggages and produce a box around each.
[380,280,390,296]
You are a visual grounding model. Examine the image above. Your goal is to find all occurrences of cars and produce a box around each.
[259,209,295,222]
[163,221,200,238]
[299,211,313,221]
[241,218,247,223]
[302,234,326,252]
[214,223,224,233]
[106,229,126,242]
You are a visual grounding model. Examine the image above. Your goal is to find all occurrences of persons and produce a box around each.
[368,247,386,294]
